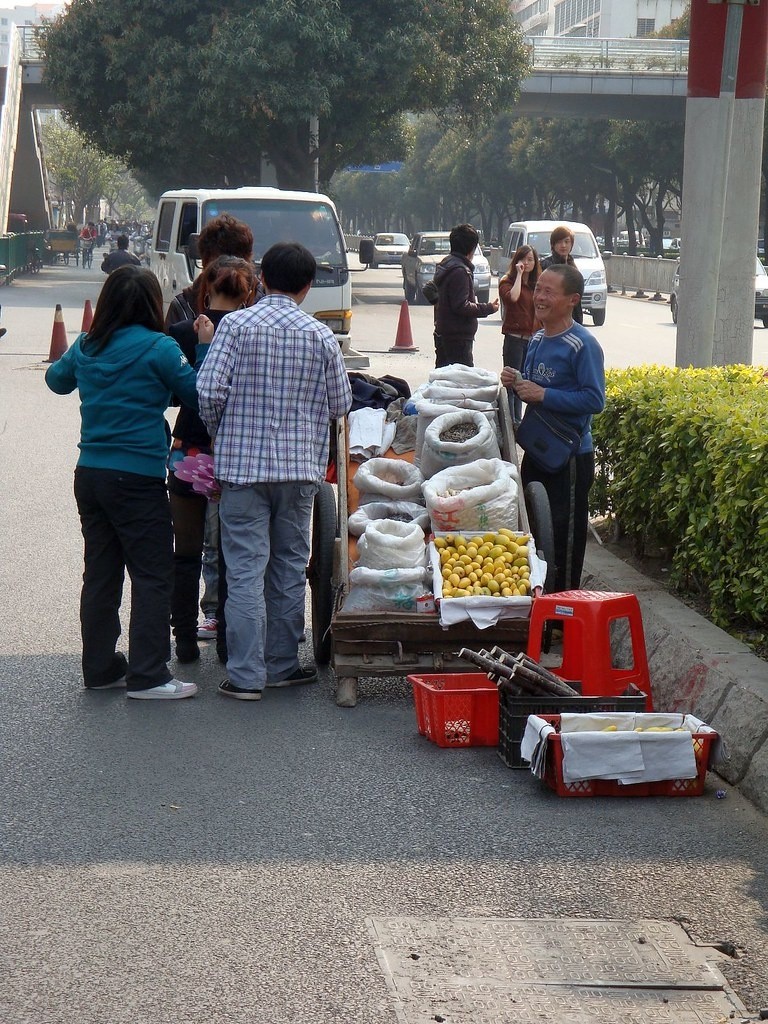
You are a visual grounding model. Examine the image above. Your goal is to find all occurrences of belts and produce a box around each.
[509,332,530,341]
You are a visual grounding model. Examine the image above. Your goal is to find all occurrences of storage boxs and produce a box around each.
[531,715,718,797]
[497,682,648,768]
[406,672,499,746]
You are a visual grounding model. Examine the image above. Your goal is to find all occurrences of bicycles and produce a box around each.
[23,245,43,275]
[77,236,96,270]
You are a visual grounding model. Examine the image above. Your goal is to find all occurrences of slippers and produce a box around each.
[549,629,563,643]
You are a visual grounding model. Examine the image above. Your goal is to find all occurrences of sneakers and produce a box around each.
[198,617,218,639]
[218,679,261,700]
[128,678,197,699]
[265,666,318,687]
[90,675,128,690]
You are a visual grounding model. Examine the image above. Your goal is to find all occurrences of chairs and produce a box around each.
[425,241,436,254]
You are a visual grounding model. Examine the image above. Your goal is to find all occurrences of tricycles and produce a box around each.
[46,228,81,267]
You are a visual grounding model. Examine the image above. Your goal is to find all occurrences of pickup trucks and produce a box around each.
[400,232,493,304]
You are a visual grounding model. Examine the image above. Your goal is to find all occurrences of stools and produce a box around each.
[526,589,654,713]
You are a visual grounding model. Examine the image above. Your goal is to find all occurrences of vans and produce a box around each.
[496,220,613,327]
[146,184,376,355]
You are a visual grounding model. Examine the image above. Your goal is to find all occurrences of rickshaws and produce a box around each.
[307,371,562,680]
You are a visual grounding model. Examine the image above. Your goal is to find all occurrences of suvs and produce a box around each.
[369,232,411,269]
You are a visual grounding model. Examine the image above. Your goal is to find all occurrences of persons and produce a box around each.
[197,242,353,698]
[423,223,499,369]
[45,266,197,699]
[541,227,583,325]
[499,245,543,429]
[101,236,141,273]
[68,217,155,259]
[163,216,262,664]
[502,265,606,641]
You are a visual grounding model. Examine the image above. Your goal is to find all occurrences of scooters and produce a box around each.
[103,232,152,279]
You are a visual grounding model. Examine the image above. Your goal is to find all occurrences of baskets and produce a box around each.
[80,240,92,249]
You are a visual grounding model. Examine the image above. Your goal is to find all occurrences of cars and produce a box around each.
[668,256,768,328]
[594,230,680,249]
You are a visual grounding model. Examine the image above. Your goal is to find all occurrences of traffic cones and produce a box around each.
[41,304,69,362]
[80,299,94,333]
[387,299,420,352]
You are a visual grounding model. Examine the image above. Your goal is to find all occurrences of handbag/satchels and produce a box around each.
[518,397,581,471]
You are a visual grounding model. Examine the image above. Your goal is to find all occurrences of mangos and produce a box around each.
[603,725,702,791]
[434,528,530,598]
